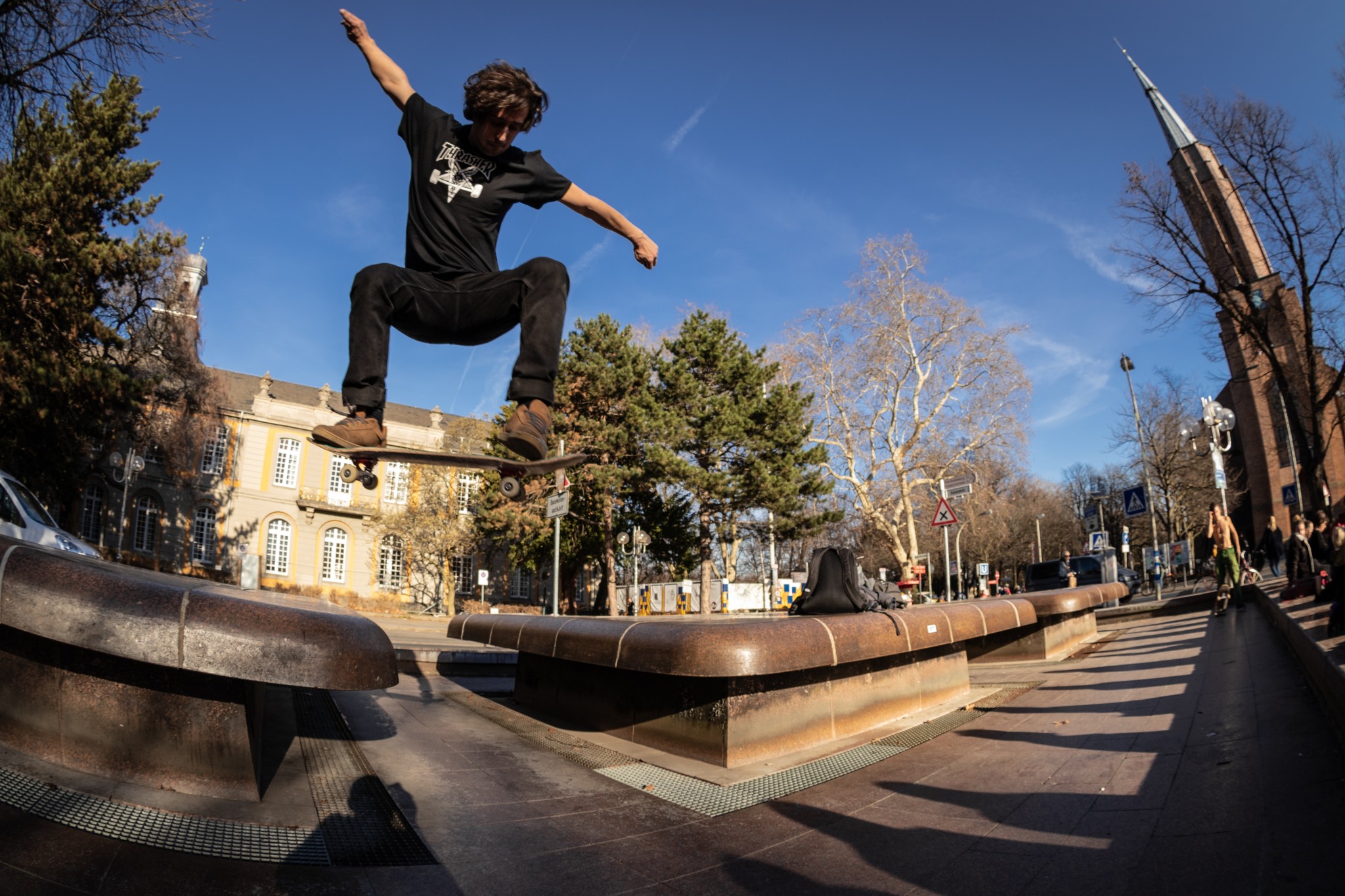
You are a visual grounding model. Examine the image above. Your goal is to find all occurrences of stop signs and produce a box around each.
[994,571,1000,580]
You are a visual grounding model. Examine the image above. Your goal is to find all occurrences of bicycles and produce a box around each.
[1140,567,1177,597]
[1193,548,1264,594]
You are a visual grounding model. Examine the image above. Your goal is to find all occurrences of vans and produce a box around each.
[1023,555,1143,605]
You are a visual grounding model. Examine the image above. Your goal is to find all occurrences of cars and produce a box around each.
[0,471,106,560]
[915,591,940,603]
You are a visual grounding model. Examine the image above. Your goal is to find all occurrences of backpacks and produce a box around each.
[788,546,869,615]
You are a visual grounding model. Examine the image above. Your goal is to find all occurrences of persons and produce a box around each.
[981,583,1026,598]
[1205,502,1246,611]
[311,8,660,461]
[627,598,635,615]
[932,590,966,603]
[1207,544,1219,574]
[1058,551,1077,588]
[1153,556,1166,589]
[1240,509,1345,639]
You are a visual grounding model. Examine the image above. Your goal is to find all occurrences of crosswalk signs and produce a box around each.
[1122,484,1149,519]
[1092,532,1108,550]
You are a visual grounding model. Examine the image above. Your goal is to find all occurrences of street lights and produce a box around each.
[1180,395,1234,518]
[1120,353,1163,601]
[1036,513,1045,564]
[109,448,145,564]
[1091,490,1110,608]
[956,509,992,601]
[617,525,651,617]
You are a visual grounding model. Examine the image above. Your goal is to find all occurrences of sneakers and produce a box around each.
[495,401,551,461]
[312,399,388,451]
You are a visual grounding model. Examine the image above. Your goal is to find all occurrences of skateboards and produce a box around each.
[306,436,587,503]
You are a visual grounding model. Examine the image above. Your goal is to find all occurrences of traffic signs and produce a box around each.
[944,472,978,488]
